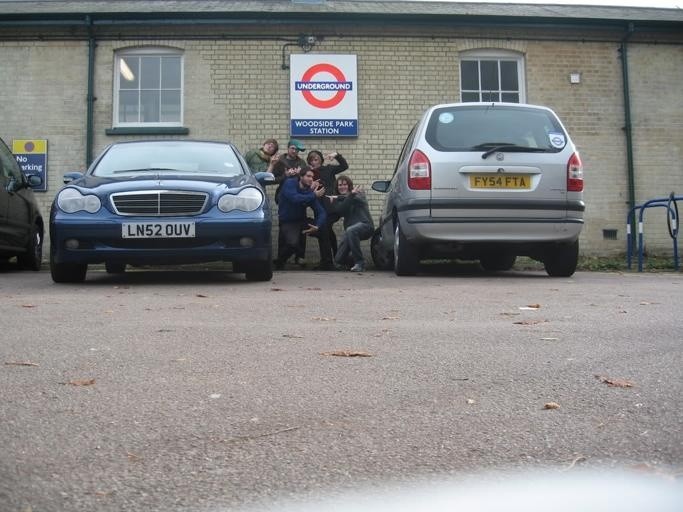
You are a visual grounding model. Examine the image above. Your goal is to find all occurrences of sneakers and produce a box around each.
[349,263,366,273]
[276,256,337,270]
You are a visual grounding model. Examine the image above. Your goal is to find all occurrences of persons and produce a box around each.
[271,138,307,266]
[292,148,348,259]
[324,175,374,272]
[272,165,337,271]
[243,136,278,192]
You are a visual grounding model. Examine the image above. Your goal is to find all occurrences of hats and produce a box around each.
[287,138,306,152]
[306,150,324,166]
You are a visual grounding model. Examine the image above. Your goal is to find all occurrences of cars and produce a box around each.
[49,138,276,283]
[370,101,586,276]
[0,137,44,271]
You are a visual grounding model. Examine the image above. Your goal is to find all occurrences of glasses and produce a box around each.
[288,146,298,151]
